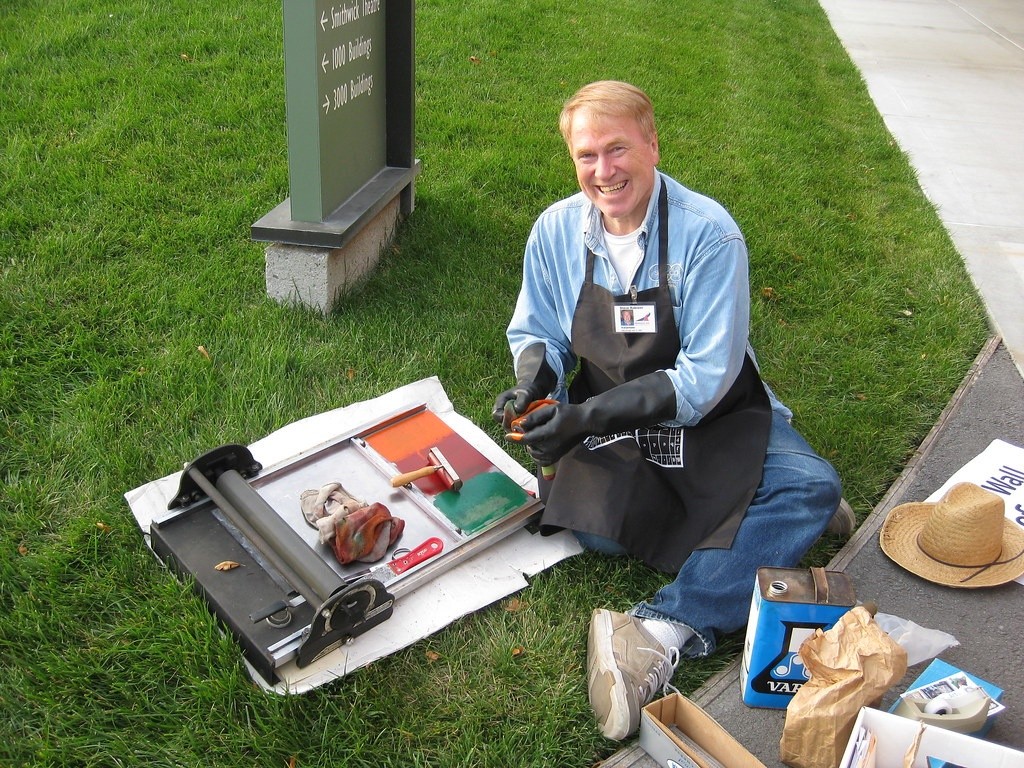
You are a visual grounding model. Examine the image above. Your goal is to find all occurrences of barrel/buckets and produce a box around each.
[737,566,856,710]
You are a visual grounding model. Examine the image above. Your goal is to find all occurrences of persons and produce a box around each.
[491,81,856,743]
[621,311,634,326]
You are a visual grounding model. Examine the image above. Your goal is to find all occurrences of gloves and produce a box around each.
[504,372,677,466]
[492,343,559,425]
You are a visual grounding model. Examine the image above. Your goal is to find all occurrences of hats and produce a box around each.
[879,482,1024,587]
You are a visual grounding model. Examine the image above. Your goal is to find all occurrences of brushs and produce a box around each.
[391,447,463,496]
[510,401,559,481]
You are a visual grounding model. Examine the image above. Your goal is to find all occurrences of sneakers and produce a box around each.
[587,608,680,741]
[826,497,855,536]
